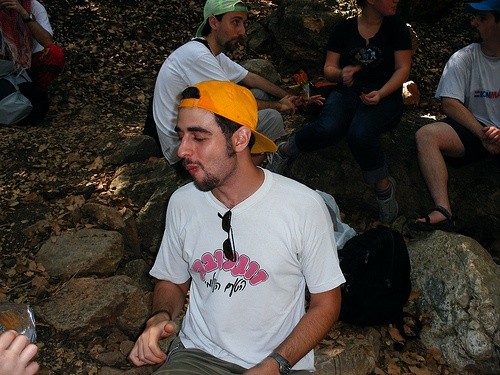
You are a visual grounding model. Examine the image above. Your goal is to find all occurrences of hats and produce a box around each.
[178,79,277,153]
[195,0,248,36]
[468,0,500,10]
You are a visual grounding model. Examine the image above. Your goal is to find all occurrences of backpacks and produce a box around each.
[0,58,34,124]
[305,223,423,352]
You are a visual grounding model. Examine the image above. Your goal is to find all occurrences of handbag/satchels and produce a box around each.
[144,95,161,148]
[315,188,357,250]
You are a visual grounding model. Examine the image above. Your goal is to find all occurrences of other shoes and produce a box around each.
[264,139,289,176]
[32,89,52,125]
[376,176,398,224]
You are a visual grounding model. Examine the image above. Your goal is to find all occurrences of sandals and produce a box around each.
[407,205,456,232]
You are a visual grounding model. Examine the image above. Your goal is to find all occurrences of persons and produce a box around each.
[153,0,326,164]
[128,81,347,375]
[407,0,500,231]
[265,1,414,224]
[0,0,65,87]
[0,329,40,375]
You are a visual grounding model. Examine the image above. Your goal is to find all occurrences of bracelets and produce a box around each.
[144,310,172,324]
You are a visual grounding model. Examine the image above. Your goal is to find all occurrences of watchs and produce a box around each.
[266,352,293,375]
[21,13,36,23]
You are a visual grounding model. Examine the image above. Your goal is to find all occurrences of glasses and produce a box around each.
[218,210,236,261]
